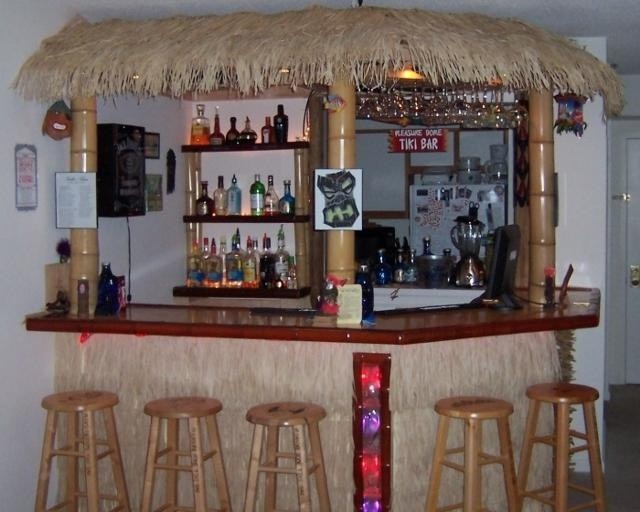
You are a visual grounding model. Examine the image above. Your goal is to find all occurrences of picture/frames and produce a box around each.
[145,132,160,159]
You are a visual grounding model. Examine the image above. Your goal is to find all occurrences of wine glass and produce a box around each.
[356,80,528,129]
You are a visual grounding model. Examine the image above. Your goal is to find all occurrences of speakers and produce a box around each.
[97,123,147,219]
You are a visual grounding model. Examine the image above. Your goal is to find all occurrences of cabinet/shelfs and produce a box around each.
[173,87,322,310]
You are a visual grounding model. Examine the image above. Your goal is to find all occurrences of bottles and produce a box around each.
[356,265,374,319]
[189,104,288,145]
[322,281,339,316]
[374,237,452,285]
[189,229,297,289]
[196,174,294,216]
[98,262,119,315]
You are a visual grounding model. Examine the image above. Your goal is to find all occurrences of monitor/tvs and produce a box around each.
[484,223,524,312]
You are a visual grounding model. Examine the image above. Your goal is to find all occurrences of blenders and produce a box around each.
[451,216,486,287]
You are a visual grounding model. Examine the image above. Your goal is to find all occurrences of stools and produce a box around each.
[141,397,233,512]
[427,397,519,512]
[33,390,133,512]
[518,383,607,512]
[244,402,332,512]
